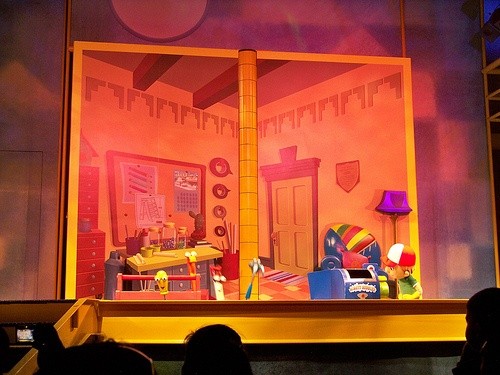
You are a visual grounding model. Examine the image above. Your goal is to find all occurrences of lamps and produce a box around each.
[376,190,414,248]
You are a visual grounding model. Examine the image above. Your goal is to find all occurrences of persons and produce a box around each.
[453,287,500,375]
[180,324,253,375]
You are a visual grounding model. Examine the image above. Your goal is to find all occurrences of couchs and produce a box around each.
[321,223,381,271]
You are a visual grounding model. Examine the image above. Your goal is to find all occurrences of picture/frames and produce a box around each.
[105,149,208,247]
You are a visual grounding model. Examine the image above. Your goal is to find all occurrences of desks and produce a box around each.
[126,247,224,297]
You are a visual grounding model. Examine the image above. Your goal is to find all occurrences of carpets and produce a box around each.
[261,269,309,286]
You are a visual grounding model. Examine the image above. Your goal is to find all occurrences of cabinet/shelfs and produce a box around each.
[77,164,106,299]
